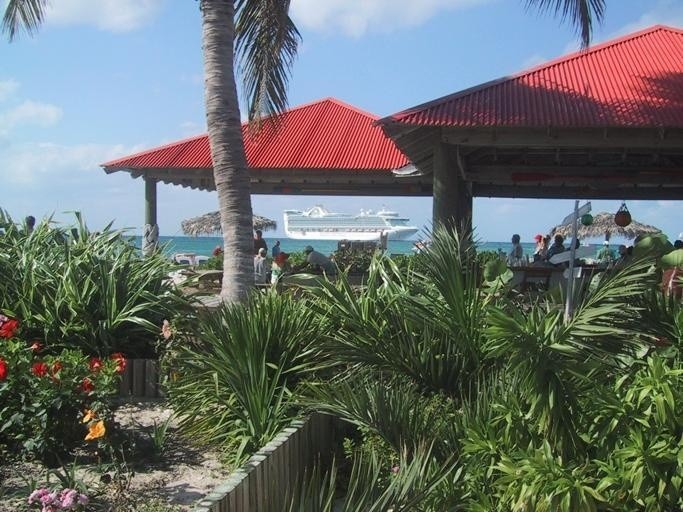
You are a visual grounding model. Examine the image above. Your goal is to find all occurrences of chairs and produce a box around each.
[520,268,552,293]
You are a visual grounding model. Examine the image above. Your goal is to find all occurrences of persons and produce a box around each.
[253,230,335,285]
[674,239,683,249]
[506,233,583,269]
[596,241,633,264]
[20,216,35,235]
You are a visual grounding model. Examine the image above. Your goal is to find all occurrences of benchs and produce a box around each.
[256,283,272,294]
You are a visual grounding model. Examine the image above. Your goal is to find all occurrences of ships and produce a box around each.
[282,202,419,243]
